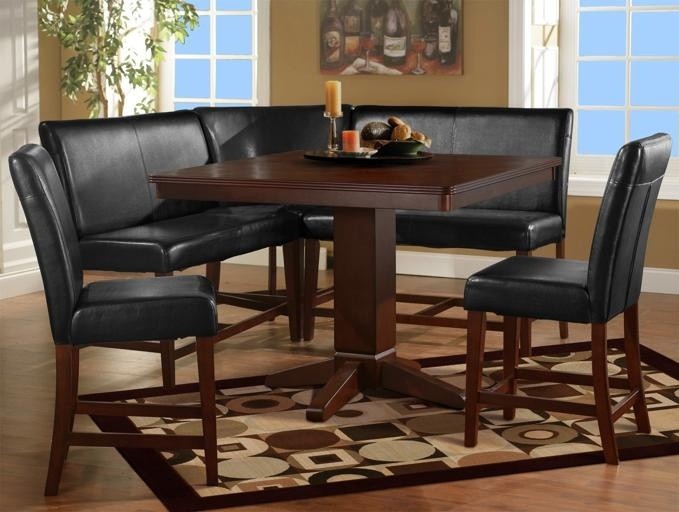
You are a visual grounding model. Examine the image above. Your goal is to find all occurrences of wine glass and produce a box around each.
[408,31,428,74]
[358,32,379,73]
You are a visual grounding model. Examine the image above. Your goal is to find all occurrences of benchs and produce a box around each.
[286,104,574,342]
[37,108,301,395]
[194,104,355,342]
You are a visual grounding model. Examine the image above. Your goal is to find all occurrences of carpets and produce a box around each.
[77,336,679,512]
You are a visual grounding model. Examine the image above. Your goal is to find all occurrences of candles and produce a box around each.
[341,131,360,152]
[325,81,342,120]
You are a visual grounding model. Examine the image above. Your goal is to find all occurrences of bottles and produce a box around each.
[366,0,407,67]
[422,1,458,65]
[322,1,363,68]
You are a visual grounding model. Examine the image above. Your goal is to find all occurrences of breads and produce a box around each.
[388,117,425,141]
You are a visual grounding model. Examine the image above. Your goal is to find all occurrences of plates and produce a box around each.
[305,151,434,162]
[334,148,378,159]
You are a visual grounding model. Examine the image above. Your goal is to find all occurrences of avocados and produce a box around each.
[361,121,392,140]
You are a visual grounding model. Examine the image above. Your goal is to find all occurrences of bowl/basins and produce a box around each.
[377,138,427,155]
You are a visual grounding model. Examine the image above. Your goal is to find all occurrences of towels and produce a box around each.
[341,56,403,76]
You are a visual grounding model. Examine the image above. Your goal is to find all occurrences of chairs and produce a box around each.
[8,142,223,497]
[461,131,672,466]
